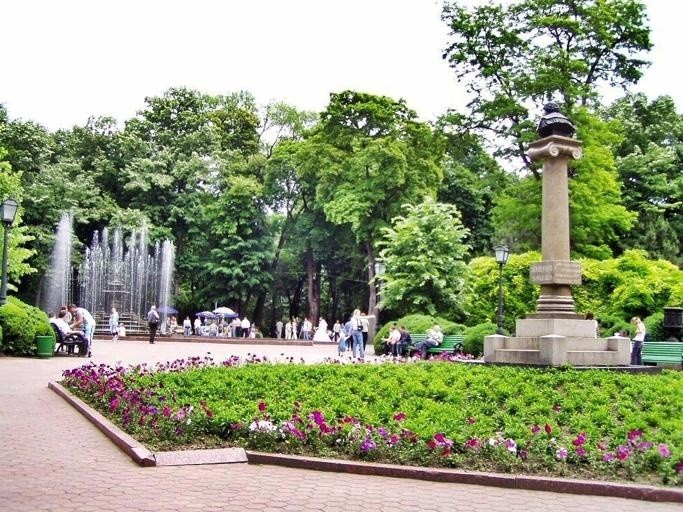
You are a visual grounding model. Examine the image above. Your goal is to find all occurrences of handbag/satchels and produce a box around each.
[148,322,158,329]
[358,326,363,331]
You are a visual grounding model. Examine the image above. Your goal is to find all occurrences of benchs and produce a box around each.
[632,341,683,365]
[403,334,464,356]
[50,322,87,357]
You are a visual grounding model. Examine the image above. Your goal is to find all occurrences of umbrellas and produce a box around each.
[157,306,179,313]
[195,306,239,319]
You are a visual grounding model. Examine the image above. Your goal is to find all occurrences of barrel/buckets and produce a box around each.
[35,335,55,357]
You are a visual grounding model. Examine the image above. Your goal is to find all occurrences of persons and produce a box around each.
[380,326,402,358]
[108,308,118,342]
[410,325,444,360]
[49,304,97,358]
[112,322,126,341]
[336,331,350,358]
[333,319,340,342]
[360,312,369,351]
[392,325,412,357]
[585,311,597,328]
[613,316,646,365]
[343,317,353,351]
[147,305,160,344]
[275,315,332,342]
[161,314,256,338]
[351,308,376,358]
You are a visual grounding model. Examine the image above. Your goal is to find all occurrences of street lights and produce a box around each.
[374,257,387,332]
[495,246,509,335]
[0,198,18,305]
